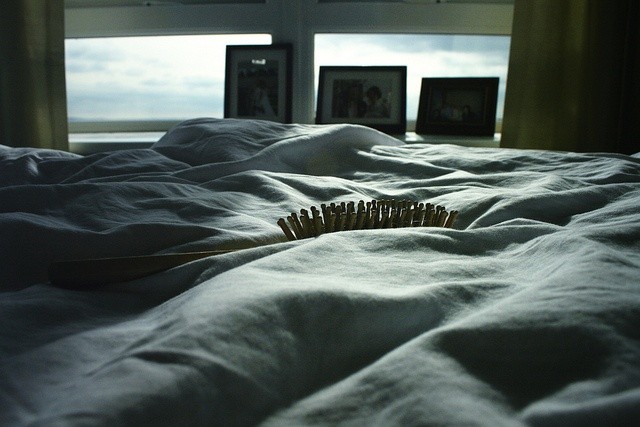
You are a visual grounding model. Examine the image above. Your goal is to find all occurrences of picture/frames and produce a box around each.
[224,43,292,127]
[315,65,406,136]
[415,77,499,138]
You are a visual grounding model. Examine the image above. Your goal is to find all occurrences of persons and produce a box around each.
[248,67,274,116]
[365,85,390,119]
[337,80,366,118]
[439,102,473,124]
